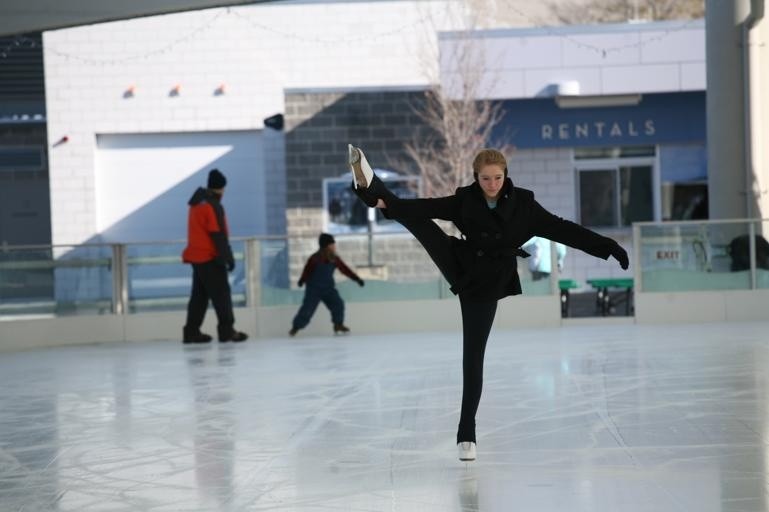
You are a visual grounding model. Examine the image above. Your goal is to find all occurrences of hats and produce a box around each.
[319,232,335,245]
[208,169,227,189]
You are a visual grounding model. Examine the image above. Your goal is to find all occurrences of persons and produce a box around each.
[182,168,249,344]
[347,144,631,461]
[287,232,367,337]
[459,460,481,512]
[188,344,237,510]
[520,235,566,281]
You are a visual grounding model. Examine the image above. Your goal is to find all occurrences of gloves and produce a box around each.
[611,243,630,270]
[226,255,235,271]
[298,279,303,286]
[353,183,379,207]
[356,280,365,287]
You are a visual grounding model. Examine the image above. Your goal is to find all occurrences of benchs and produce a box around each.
[559,278,633,318]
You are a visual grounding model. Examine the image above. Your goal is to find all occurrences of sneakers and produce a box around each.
[219,331,247,342]
[289,327,297,334]
[458,441,476,461]
[353,148,373,187]
[333,325,351,331]
[184,332,212,344]
